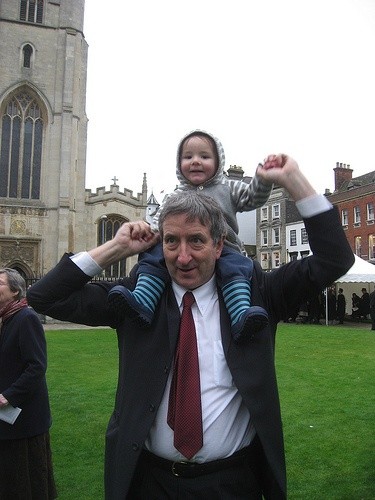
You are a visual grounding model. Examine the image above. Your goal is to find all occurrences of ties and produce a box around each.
[166,292,203,460]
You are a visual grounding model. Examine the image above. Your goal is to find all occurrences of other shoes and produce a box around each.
[230,305,269,343]
[106,285,153,331]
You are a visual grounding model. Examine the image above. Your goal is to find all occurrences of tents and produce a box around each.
[303,252,375,326]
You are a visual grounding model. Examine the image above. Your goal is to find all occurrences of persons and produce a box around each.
[281,283,375,332]
[0,268,58,499]
[106,128,278,347]
[26,152,355,500]
